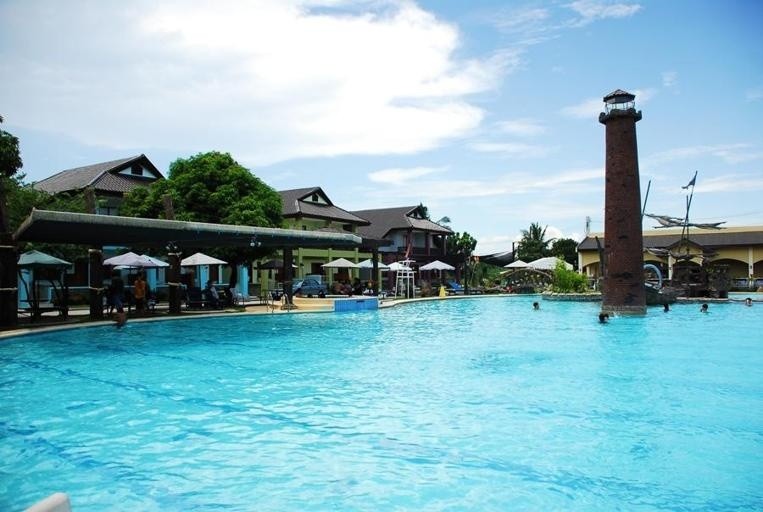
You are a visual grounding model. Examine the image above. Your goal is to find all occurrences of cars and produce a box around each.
[272,277,328,300]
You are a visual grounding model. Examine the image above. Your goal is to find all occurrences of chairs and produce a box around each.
[187,287,209,311]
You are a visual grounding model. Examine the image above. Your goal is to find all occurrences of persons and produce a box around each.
[599,313,609,325]
[532,302,539,309]
[744,298,752,306]
[700,304,708,313]
[419,278,454,296]
[664,303,671,312]
[326,277,398,297]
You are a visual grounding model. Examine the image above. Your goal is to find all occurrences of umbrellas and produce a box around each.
[258,259,299,299]
[503,256,574,272]
[321,257,455,292]
[101,251,229,312]
[17,249,72,308]
